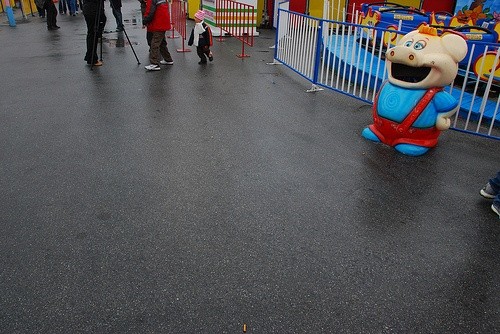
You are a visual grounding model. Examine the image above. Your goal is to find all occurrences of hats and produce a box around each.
[194,10,205,20]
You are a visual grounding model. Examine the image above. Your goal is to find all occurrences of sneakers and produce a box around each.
[160,59,174,64]
[145,64,160,70]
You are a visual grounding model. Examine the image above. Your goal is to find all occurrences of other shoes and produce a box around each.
[209,56,213,61]
[491,199,500,216]
[198,60,207,64]
[94,60,102,65]
[479,182,498,199]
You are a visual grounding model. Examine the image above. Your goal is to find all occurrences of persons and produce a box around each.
[41,0,60,31]
[479,170,500,217]
[109,0,123,32]
[141,0,174,70]
[188,10,213,65]
[59,0,67,15]
[81,0,107,65]
[138,0,163,61]
[66,0,83,17]
[34,0,45,18]
